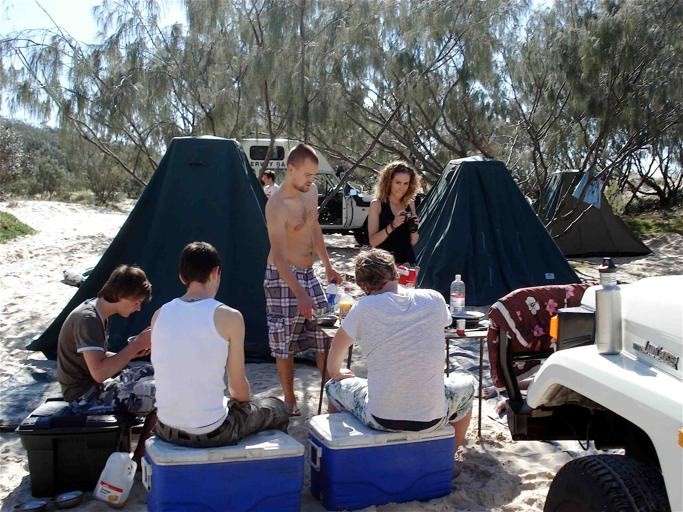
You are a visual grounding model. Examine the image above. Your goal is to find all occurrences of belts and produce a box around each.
[158,420,229,440]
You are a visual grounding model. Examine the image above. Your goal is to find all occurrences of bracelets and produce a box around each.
[385,225,390,236]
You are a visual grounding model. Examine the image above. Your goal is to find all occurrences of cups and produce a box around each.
[457,320,465,336]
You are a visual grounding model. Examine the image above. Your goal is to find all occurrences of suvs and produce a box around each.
[495,274,683,512]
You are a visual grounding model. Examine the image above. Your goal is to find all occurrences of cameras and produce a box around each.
[400,212,418,233]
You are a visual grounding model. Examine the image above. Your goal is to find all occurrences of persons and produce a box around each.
[262,145,342,416]
[324,248,475,479]
[261,170,281,199]
[484,377,538,415]
[150,239,289,448]
[55,262,159,470]
[368,160,421,277]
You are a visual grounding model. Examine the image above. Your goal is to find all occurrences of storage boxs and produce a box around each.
[17,390,131,498]
[308,412,455,512]
[141,427,306,512]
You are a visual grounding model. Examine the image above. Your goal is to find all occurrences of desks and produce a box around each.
[317,312,489,438]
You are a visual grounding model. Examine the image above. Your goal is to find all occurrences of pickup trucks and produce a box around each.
[242,137,373,244]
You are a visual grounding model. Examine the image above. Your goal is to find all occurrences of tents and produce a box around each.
[415,154,582,307]
[530,170,653,255]
[24,136,318,363]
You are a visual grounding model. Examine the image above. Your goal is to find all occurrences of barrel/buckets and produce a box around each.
[92,452,138,507]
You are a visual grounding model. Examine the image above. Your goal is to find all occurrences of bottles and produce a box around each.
[596,258,623,355]
[339,288,352,319]
[325,280,337,310]
[449,275,465,314]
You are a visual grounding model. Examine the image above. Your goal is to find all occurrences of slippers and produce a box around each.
[285,403,301,416]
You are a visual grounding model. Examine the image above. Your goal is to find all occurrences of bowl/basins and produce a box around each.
[14,501,48,512]
[451,311,485,328]
[128,335,149,352]
[315,315,338,326]
[51,491,83,509]
